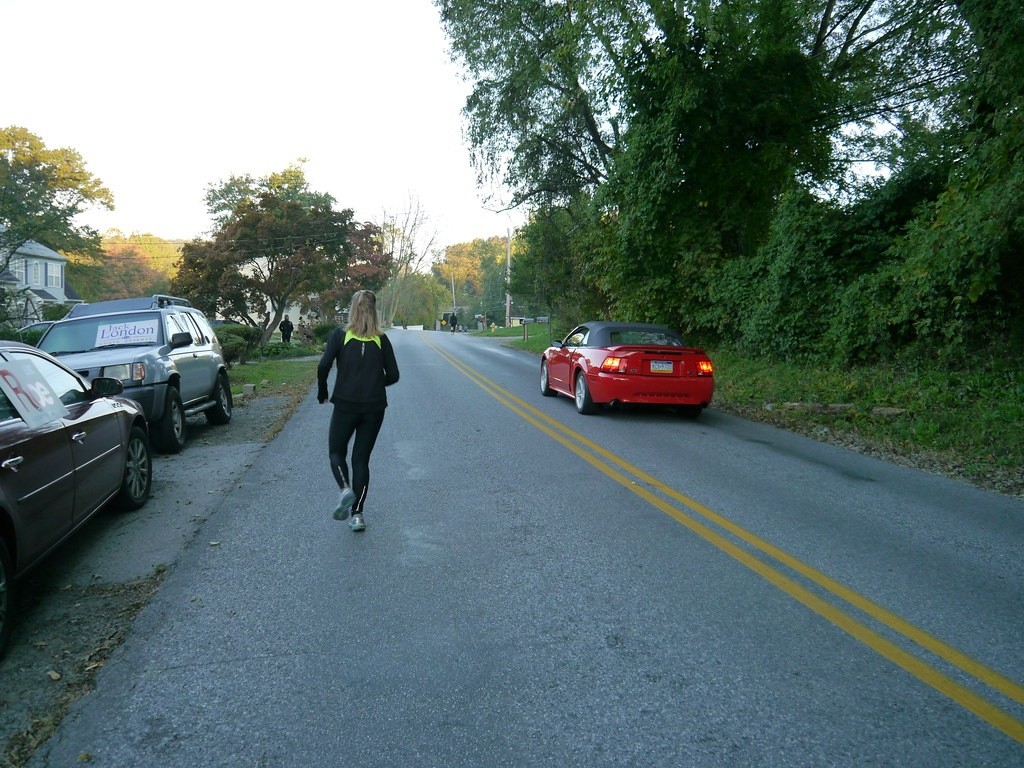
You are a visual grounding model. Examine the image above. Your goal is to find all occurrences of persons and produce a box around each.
[449,311,458,336]
[298,310,322,333]
[279,313,294,344]
[315,288,402,532]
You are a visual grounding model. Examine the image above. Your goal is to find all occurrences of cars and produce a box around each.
[0,339,152,660]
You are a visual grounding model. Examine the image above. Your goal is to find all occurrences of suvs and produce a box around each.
[33,295,235,454]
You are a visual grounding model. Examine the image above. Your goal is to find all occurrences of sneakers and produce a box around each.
[332,487,355,520]
[346,513,365,531]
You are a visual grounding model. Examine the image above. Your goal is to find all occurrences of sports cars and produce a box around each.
[537,321,715,420]
